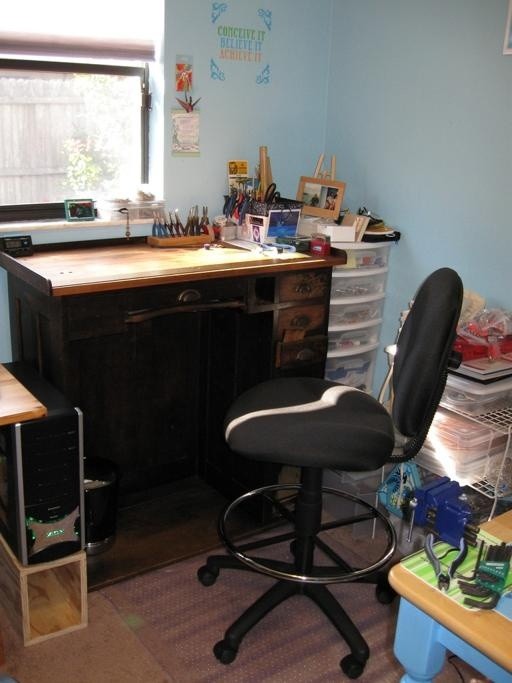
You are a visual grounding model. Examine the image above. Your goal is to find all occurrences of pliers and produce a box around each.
[425,532,467,591]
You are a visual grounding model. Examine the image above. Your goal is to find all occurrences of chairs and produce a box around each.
[196,268,467,678]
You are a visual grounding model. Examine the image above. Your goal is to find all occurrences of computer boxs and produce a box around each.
[0,360,86,566]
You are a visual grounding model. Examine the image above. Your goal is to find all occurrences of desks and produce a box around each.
[0,230,383,592]
[0,358,90,648]
[389,510,511,681]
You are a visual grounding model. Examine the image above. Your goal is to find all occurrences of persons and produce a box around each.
[309,192,319,207]
[327,190,337,209]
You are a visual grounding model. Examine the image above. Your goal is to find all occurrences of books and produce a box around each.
[449,352,511,384]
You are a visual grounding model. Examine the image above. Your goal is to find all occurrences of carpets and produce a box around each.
[0,525,489,681]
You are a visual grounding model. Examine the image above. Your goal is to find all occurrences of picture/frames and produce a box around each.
[64,198,94,222]
[296,174,345,221]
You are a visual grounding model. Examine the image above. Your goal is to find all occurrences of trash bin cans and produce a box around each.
[78,457,119,556]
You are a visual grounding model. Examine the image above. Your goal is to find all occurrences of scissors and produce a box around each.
[153,205,210,239]
[264,183,280,207]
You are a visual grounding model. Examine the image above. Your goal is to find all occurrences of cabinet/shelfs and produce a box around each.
[326,241,399,522]
[375,349,512,546]
